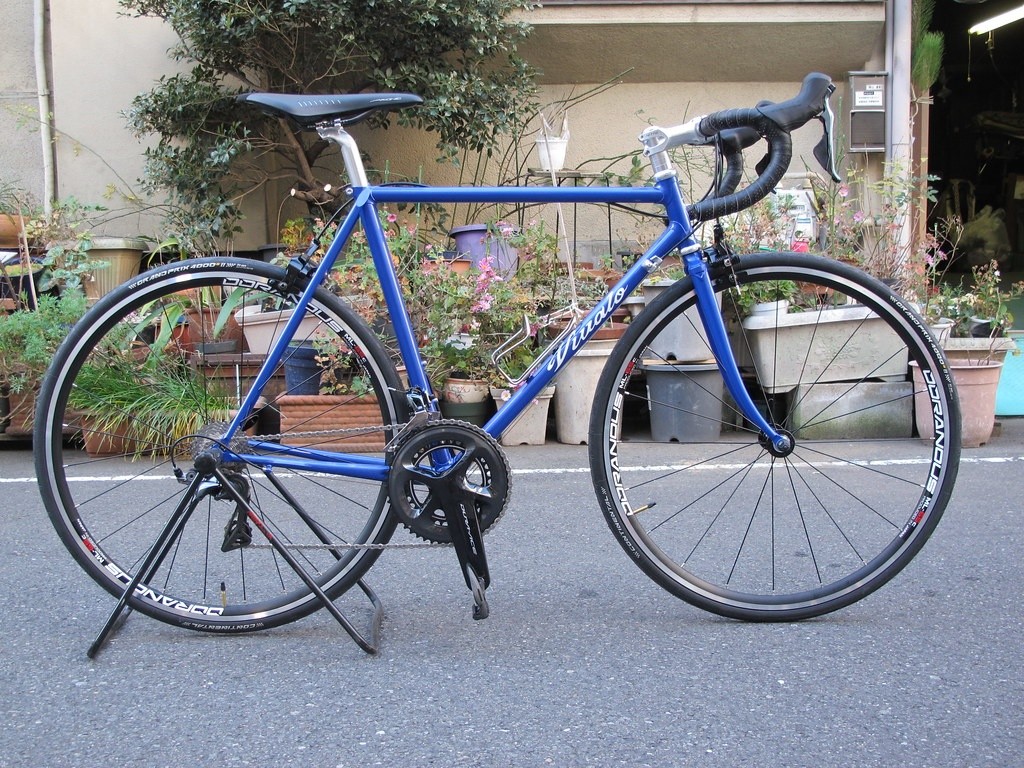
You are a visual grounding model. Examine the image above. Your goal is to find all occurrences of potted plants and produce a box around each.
[0,1,1024,449]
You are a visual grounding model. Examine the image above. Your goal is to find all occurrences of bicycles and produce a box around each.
[36,70,962,666]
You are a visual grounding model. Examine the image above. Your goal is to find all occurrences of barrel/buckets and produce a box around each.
[637,357,723,443]
[547,338,625,445]
